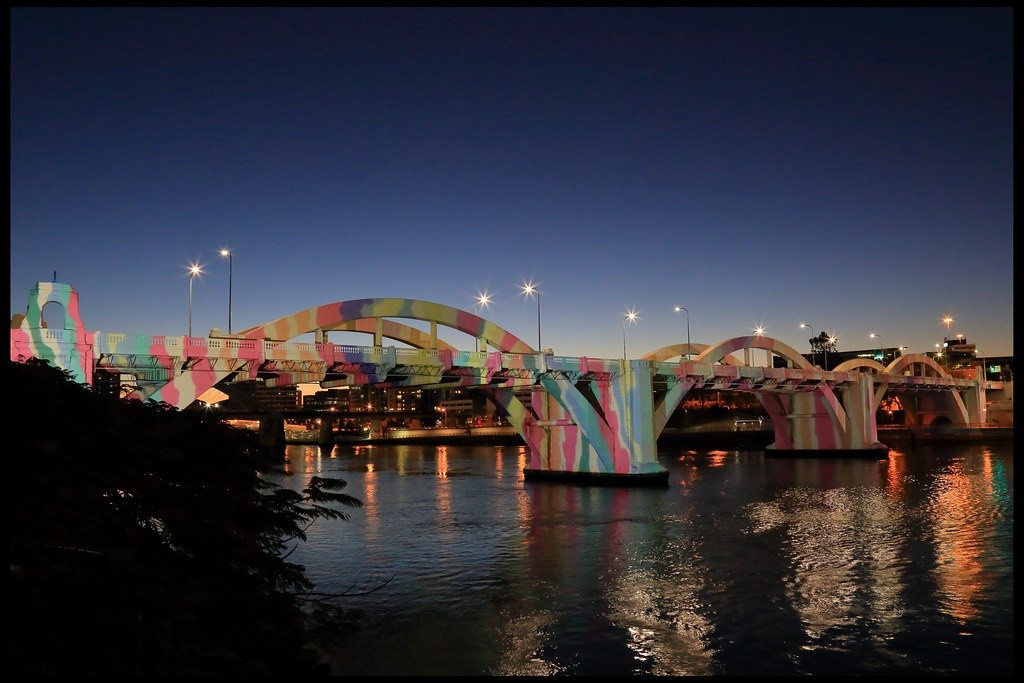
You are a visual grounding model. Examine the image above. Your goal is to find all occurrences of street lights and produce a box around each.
[675,307,690,361]
[475,298,487,352]
[944,318,952,332]
[894,346,907,360]
[221,250,232,334]
[438,407,447,426]
[623,313,634,360]
[975,350,986,380]
[526,287,541,353]
[944,342,948,372]
[189,267,199,338]
[871,334,883,365]
[752,329,762,368]
[824,338,834,371]
[801,324,815,367]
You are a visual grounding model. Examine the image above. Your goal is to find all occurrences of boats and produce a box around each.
[333,426,371,442]
